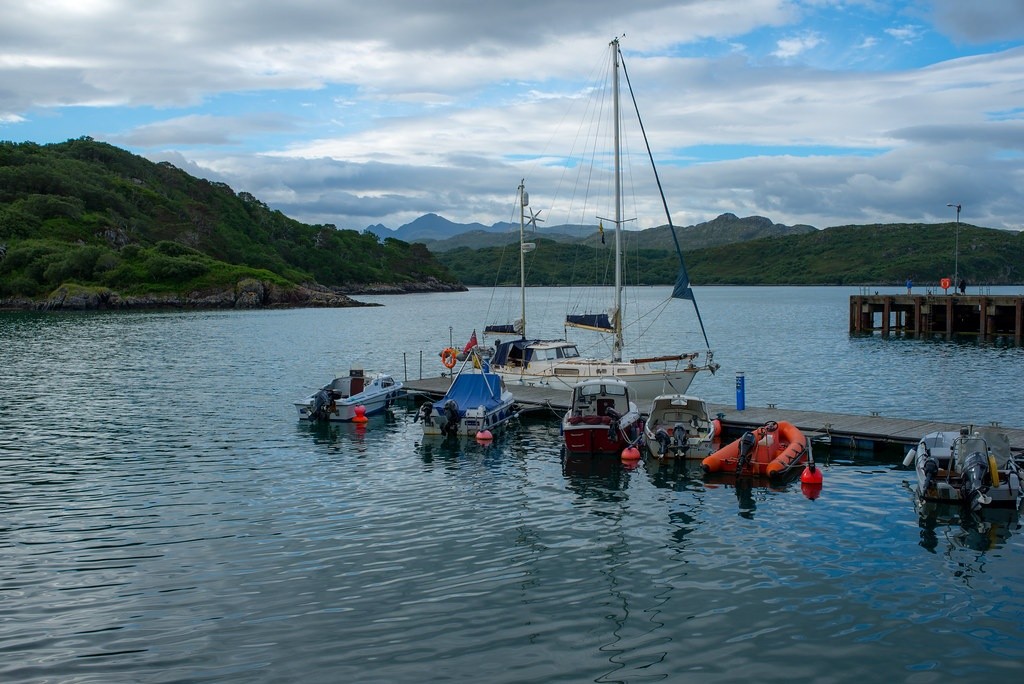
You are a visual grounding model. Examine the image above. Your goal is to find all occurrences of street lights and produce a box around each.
[946,203,961,296]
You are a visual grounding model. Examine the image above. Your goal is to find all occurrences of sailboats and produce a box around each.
[428,30,721,396]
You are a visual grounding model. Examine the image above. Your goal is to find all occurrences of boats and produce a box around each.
[645,394,718,461]
[563,379,641,455]
[704,420,807,475]
[293,367,402,421]
[912,423,1021,507]
[421,348,517,439]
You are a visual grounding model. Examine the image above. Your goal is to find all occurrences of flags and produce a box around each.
[600,221,605,244]
[463,329,478,354]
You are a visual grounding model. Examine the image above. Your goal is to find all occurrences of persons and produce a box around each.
[905,277,912,294]
[958,279,966,296]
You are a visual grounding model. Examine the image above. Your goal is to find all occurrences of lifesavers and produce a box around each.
[989,455,1000,488]
[442,349,455,368]
